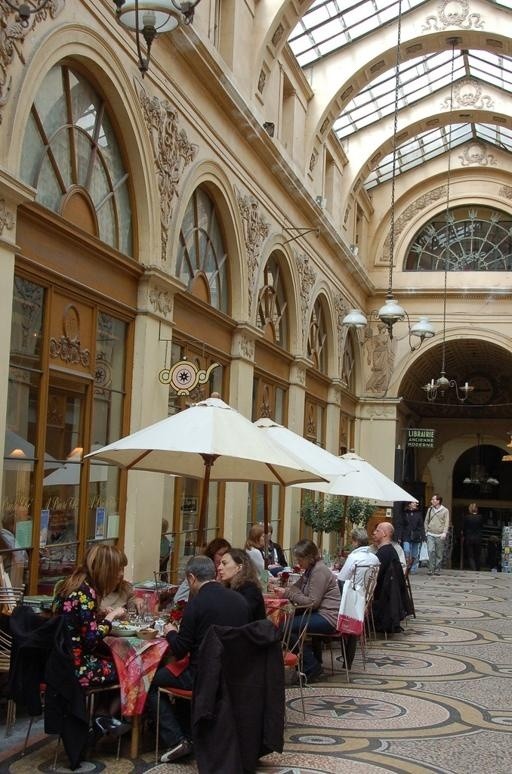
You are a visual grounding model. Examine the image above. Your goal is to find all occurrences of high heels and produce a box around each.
[93,714,131,744]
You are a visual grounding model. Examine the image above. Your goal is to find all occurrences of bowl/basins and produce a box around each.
[135,628,159,641]
[110,625,137,637]
[144,583,153,588]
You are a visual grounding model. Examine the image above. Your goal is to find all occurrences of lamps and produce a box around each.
[343,0,435,352]
[418,35,475,402]
[462,434,500,493]
[115,0,201,80]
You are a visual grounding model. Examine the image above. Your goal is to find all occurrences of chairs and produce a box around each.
[152,570,184,609]
[265,556,417,719]
[0,584,29,734]
[0,605,124,774]
[155,619,286,773]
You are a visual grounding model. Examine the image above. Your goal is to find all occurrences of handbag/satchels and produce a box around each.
[285,665,297,685]
[410,528,422,542]
[335,579,366,635]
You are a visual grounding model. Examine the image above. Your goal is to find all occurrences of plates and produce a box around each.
[283,570,293,573]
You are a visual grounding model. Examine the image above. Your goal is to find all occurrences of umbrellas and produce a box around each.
[84,391,331,559]
[253,417,360,571]
[43,441,108,500]
[3,426,67,471]
[289,449,420,558]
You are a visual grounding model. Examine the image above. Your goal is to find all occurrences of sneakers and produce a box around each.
[427,570,433,575]
[160,736,195,763]
[434,570,440,575]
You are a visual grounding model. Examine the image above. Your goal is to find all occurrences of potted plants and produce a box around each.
[335,542,355,558]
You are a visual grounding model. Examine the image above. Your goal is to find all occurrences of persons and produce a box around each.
[148,537,266,763]
[335,523,407,633]
[461,503,482,571]
[53,543,144,738]
[397,502,427,575]
[424,494,449,576]
[2,503,75,573]
[246,525,341,684]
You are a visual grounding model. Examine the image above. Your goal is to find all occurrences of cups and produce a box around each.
[284,566,291,571]
[153,621,166,637]
